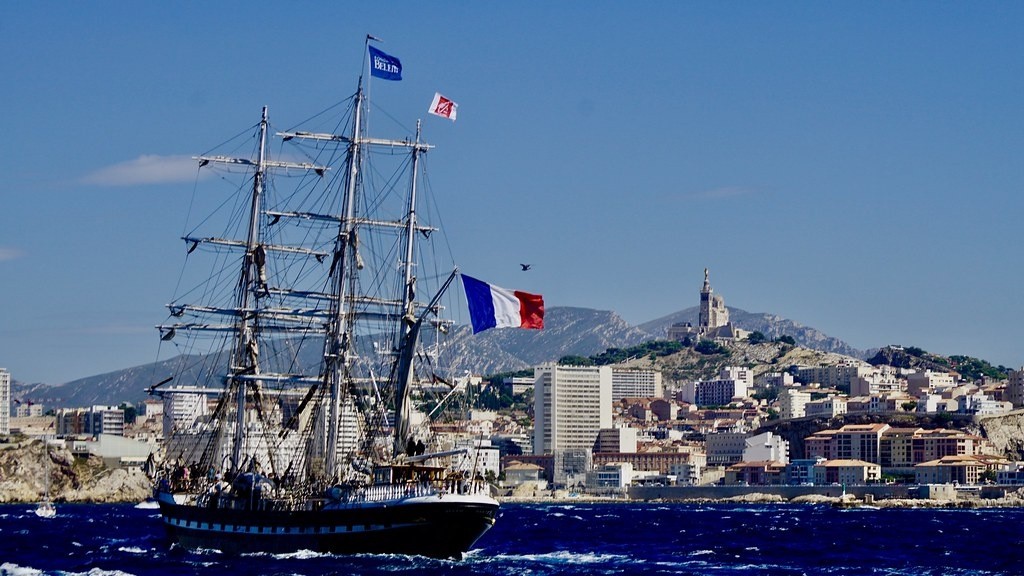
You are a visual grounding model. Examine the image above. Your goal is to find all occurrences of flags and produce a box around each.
[459,273,545,335]
[428,91,459,122]
[368,44,402,82]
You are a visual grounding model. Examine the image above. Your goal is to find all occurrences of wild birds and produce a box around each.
[520,263,531,271]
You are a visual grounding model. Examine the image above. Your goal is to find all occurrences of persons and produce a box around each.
[172,461,482,493]
[406,437,415,457]
[416,440,425,456]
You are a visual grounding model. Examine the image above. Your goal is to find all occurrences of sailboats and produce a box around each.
[142,31,505,561]
